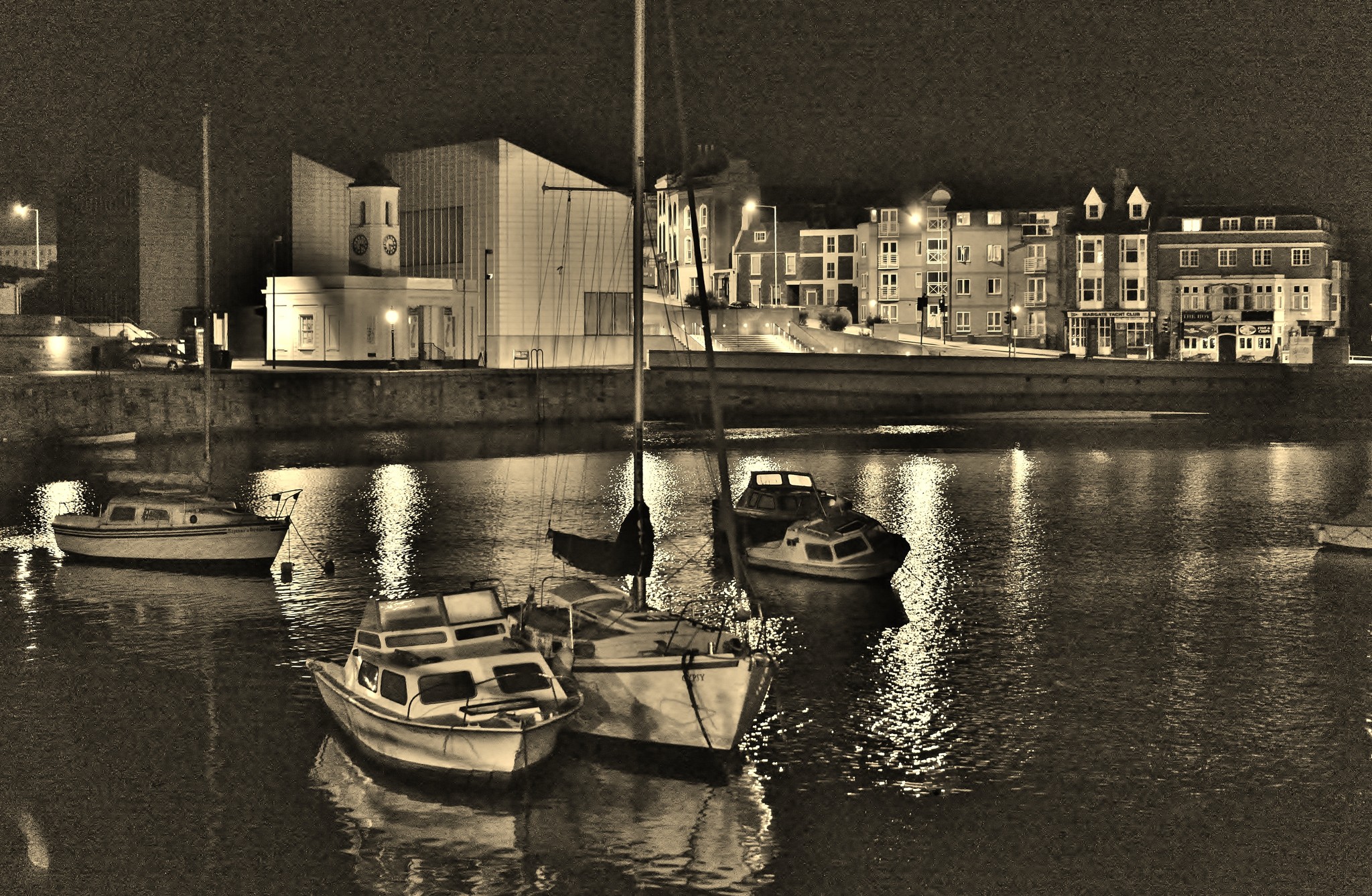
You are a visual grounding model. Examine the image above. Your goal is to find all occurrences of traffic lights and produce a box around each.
[1178,321,1186,339]
[1161,316,1171,334]
[938,297,944,312]
[1002,311,1011,325]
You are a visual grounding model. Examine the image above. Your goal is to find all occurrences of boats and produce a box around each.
[703,468,855,534]
[475,0,776,754]
[61,428,134,444]
[55,473,337,581]
[302,577,581,783]
[742,505,911,589]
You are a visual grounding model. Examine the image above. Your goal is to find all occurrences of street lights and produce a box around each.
[272,234,280,368]
[748,201,777,336]
[386,306,400,371]
[869,299,878,337]
[482,247,494,366]
[15,203,40,271]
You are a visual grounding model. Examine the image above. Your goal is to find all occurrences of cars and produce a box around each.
[124,344,188,373]
